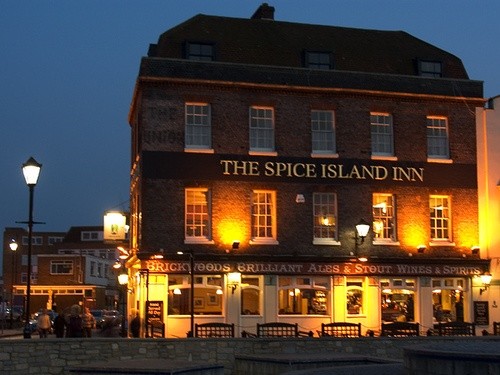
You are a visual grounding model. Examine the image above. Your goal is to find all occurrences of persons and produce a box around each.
[455,296,464,323]
[130,311,141,338]
[66,305,84,338]
[37,309,51,339]
[53,312,67,338]
[406,297,414,321]
[82,307,96,338]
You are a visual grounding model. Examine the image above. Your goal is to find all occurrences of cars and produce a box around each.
[29,308,59,333]
[91,310,123,329]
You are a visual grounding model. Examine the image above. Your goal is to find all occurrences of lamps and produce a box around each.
[354,218,370,246]
[228,264,242,294]
[216,282,223,294]
[174,283,182,294]
[477,266,493,294]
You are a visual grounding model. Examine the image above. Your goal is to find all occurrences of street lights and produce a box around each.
[14,156,47,339]
